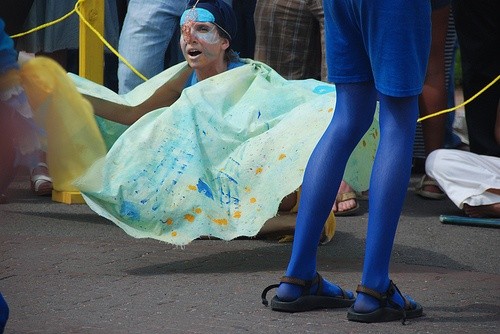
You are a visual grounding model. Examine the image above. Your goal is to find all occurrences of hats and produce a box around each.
[186,0,234,41]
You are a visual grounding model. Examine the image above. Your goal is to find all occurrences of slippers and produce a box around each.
[332,191,360,215]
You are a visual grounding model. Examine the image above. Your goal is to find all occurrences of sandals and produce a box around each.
[30,161,53,194]
[261,273,357,312]
[347,280,424,320]
[415,175,446,198]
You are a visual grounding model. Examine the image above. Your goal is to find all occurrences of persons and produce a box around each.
[0,0,500,218]
[261,0,431,326]
[81,0,338,246]
